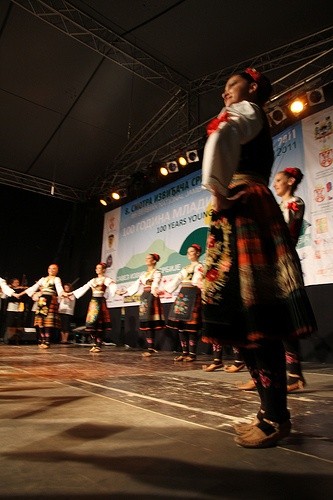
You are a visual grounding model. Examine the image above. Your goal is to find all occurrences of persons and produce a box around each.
[63,263,124,353]
[0,277,20,346]
[202,336,244,374]
[200,69,291,447]
[121,253,163,356]
[5,280,28,341]
[236,167,306,391]
[58,282,75,345]
[106,254,113,267]
[108,234,114,249]
[155,244,205,362]
[107,216,115,229]
[18,263,68,349]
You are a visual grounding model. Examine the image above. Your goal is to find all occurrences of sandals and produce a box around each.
[239,378,257,390]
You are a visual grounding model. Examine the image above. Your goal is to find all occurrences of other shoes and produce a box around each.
[186,357,196,362]
[287,380,304,392]
[224,363,245,373]
[90,347,95,352]
[43,345,48,349]
[142,352,151,357]
[174,355,183,361]
[93,347,102,352]
[233,421,257,435]
[234,420,292,447]
[203,362,224,372]
[0,338,7,346]
[38,345,44,348]
